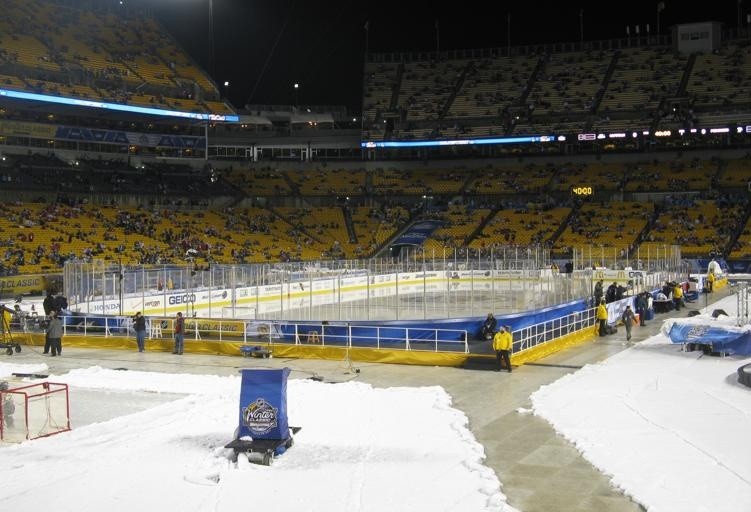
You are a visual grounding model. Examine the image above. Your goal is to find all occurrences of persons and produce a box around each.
[0,0,751,373]
